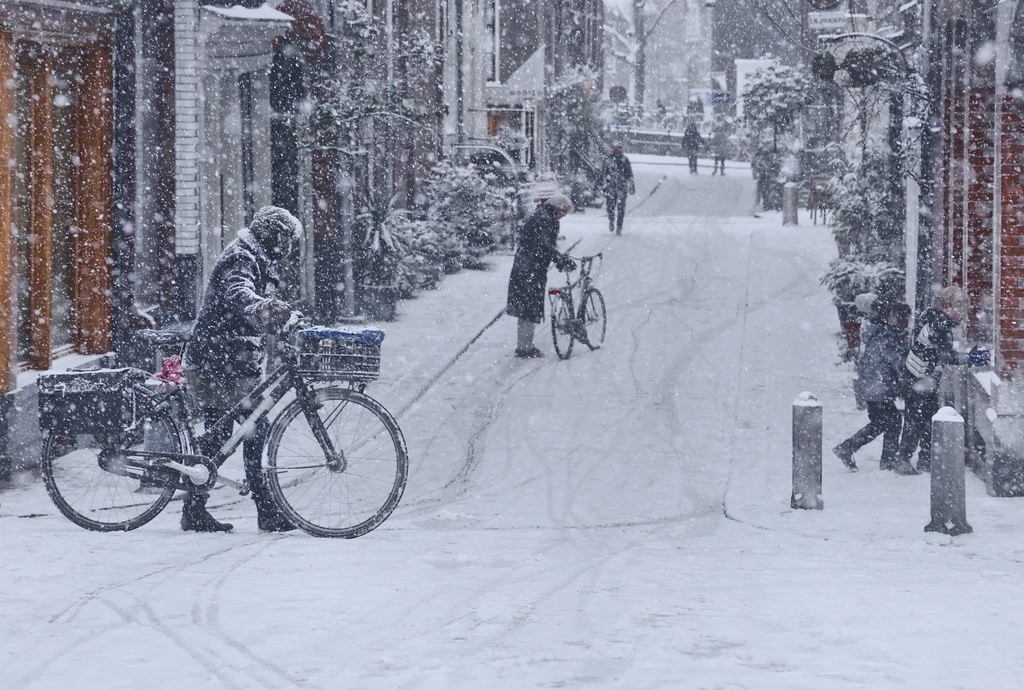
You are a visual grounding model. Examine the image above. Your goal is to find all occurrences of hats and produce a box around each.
[855,293,879,315]
[254,205,303,244]
[547,194,573,211]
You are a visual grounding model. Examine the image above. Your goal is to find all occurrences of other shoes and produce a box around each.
[917,459,931,473]
[833,441,860,472]
[515,348,545,358]
[182,509,234,532]
[880,460,898,470]
[259,516,296,532]
[617,229,622,236]
[896,459,919,474]
[610,221,614,231]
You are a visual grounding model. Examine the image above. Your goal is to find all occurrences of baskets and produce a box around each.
[300,331,382,383]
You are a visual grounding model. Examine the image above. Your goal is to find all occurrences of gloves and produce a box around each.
[270,300,292,323]
[968,344,991,366]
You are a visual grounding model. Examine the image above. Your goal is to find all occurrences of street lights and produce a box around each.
[818,33,922,345]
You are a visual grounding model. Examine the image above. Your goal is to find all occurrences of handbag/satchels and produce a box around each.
[852,378,867,410]
[37,369,134,435]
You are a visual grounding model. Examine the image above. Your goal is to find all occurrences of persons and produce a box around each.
[687,97,704,114]
[180,206,303,533]
[594,143,634,236]
[681,124,727,176]
[750,146,770,206]
[507,194,572,359]
[656,98,666,114]
[832,286,991,476]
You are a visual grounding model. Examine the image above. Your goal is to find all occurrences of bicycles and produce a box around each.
[41,284,408,539]
[548,253,607,360]
